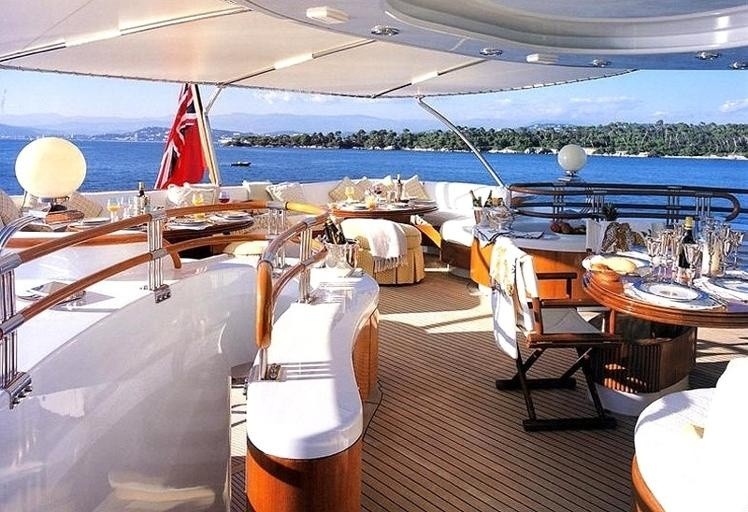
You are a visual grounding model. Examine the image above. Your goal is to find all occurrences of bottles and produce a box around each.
[394,172,404,202]
[469,189,496,207]
[134,181,147,220]
[324,217,347,245]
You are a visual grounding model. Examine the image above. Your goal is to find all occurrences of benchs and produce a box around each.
[248,264,379,512]
[468,221,672,306]
[630,357,748,511]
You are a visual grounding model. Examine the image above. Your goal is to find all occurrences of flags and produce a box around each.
[153,83,208,190]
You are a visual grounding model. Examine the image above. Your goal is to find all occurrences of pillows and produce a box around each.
[327,175,431,205]
[0,188,103,226]
[243,180,306,205]
[166,181,219,210]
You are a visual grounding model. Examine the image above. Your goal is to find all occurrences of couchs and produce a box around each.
[0,179,517,283]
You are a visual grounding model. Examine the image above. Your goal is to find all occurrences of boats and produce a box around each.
[229,161,251,167]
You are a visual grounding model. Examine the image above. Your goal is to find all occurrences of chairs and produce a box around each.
[491,234,617,432]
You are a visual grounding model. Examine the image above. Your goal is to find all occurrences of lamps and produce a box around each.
[15,136,86,214]
[555,144,590,182]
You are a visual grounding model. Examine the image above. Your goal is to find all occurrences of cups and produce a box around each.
[218,191,231,203]
[191,193,205,206]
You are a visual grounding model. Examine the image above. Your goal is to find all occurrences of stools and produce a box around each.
[353,221,424,285]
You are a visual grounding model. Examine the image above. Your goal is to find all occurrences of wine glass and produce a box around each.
[642,214,745,288]
[344,186,354,203]
[107,198,120,222]
[120,197,132,220]
[372,183,384,205]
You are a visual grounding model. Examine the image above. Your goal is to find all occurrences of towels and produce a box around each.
[366,219,408,272]
[489,236,541,362]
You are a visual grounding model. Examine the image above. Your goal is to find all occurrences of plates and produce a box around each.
[629,277,710,306]
[704,272,748,294]
